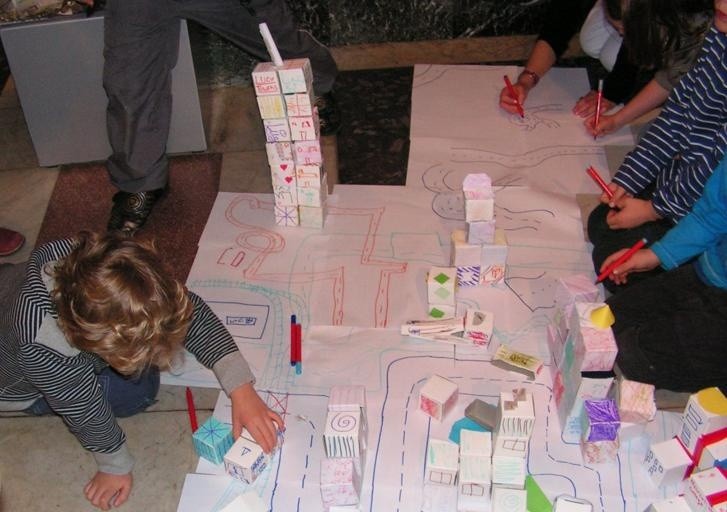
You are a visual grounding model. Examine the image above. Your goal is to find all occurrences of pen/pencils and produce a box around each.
[593,80,603,141]
[185,388,199,433]
[503,75,525,117]
[586,166,615,199]
[595,238,648,285]
[290,315,301,376]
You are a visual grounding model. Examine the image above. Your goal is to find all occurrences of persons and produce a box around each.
[0,222,285,512]
[583,0,715,137]
[499,0,654,118]
[585,0,727,296]
[604,151,727,395]
[99,0,342,241]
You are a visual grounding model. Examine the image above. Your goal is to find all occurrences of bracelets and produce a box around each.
[519,70,539,86]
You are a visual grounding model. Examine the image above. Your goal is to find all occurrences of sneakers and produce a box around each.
[103,181,171,240]
[312,84,343,138]
